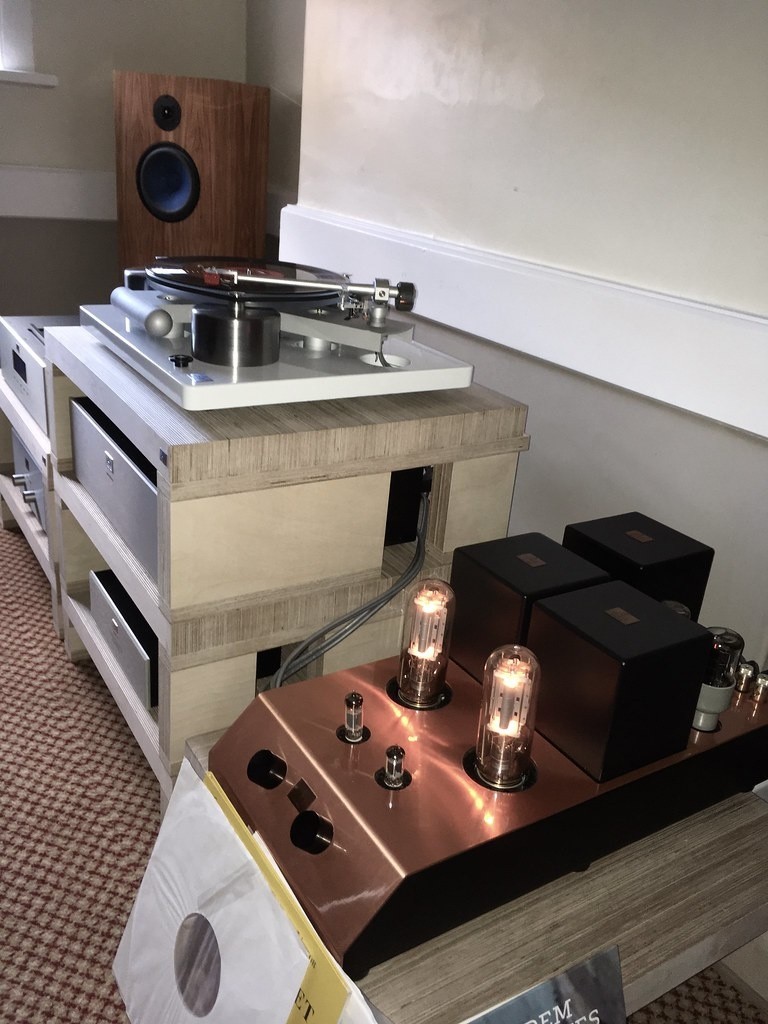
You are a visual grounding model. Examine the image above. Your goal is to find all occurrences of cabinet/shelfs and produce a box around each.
[0,313,532,830]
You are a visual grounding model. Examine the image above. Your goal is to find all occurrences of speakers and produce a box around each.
[113,70,271,283]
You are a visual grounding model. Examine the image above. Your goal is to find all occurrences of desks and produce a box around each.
[184,726,768,1024]
